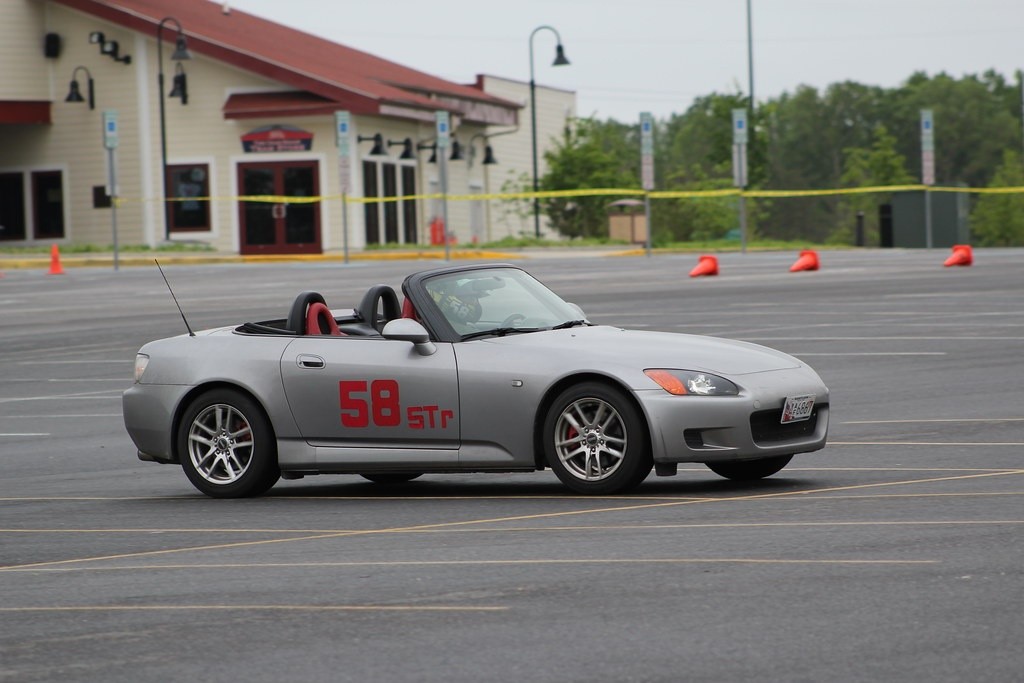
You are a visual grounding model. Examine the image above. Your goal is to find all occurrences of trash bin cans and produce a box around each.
[605,199,648,244]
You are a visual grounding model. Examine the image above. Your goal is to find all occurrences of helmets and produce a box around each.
[433,277,490,324]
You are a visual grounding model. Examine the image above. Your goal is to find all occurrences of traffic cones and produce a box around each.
[45,242,64,276]
[789,248,820,274]
[944,244,974,268]
[688,255,720,279]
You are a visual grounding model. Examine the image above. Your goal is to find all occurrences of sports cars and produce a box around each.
[122,263,830,499]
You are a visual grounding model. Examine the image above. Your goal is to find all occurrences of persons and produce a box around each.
[414,281,483,340]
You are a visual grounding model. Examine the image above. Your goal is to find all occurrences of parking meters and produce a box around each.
[103,110,118,273]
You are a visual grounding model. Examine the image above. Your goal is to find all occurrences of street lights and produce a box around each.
[529,24,573,239]
[159,15,195,244]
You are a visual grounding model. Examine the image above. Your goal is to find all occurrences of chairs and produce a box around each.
[355,283,403,335]
[286,291,346,338]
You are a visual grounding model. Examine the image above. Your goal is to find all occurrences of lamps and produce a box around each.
[387,138,417,160]
[417,142,441,162]
[171,63,190,105]
[89,32,131,64]
[64,66,96,110]
[469,136,498,164]
[450,141,463,160]
[358,133,389,156]
